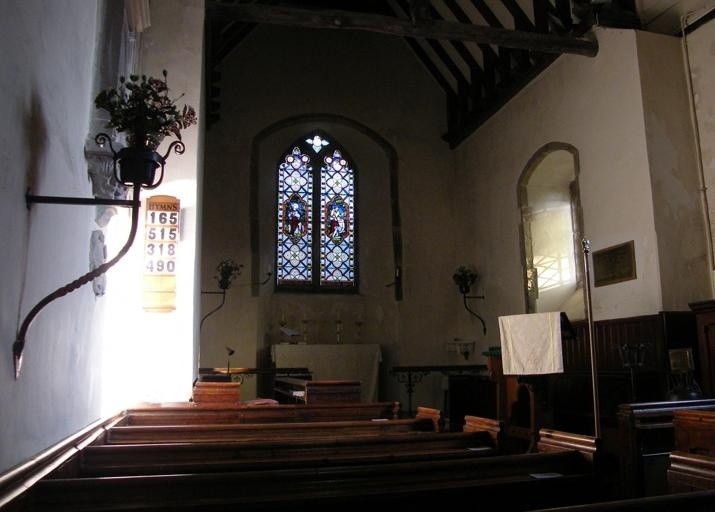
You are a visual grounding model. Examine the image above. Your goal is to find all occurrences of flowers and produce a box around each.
[214,259,244,289]
[453,266,477,293]
[93,69,198,184]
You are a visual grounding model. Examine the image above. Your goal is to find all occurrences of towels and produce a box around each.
[497,311,564,375]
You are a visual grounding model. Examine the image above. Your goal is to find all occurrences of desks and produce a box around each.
[274,376,361,404]
[271,342,384,402]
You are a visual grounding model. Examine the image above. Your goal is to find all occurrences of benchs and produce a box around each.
[570,316,644,413]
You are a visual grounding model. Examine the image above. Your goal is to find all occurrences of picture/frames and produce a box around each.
[592,240,636,287]
